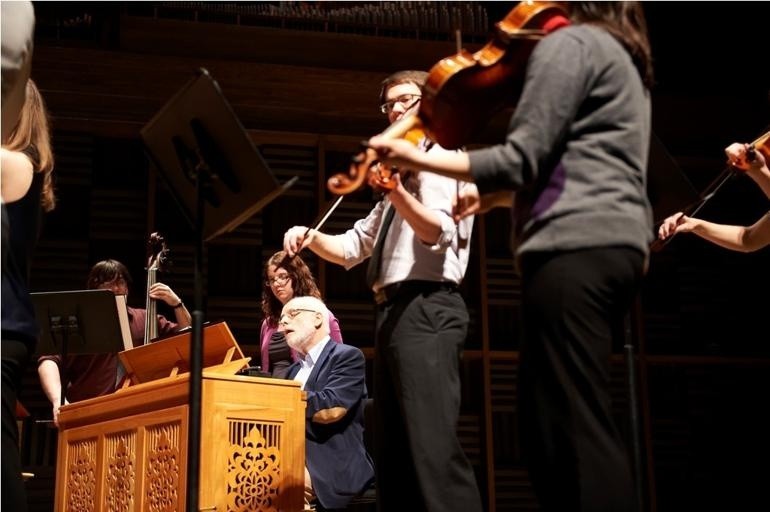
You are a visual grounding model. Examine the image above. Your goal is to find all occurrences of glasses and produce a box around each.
[265,273,291,286]
[279,308,318,321]
[381,92,421,114]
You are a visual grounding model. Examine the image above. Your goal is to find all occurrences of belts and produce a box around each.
[372,279,451,308]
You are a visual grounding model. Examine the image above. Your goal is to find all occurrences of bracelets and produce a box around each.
[173,300,183,310]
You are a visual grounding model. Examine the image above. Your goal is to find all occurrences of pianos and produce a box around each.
[53,324,309,511]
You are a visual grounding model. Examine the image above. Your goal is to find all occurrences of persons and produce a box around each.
[368,1,655,511]
[658,142,770,253]
[272,295,376,512]
[260,250,344,373]
[282,68,482,511]
[85,259,192,397]
[0,76,69,429]
[0,1,39,512]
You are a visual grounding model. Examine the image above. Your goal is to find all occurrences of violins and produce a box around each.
[328,1,569,194]
[729,134,770,169]
[367,129,430,192]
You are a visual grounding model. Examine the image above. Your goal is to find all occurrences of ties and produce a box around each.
[367,169,411,283]
